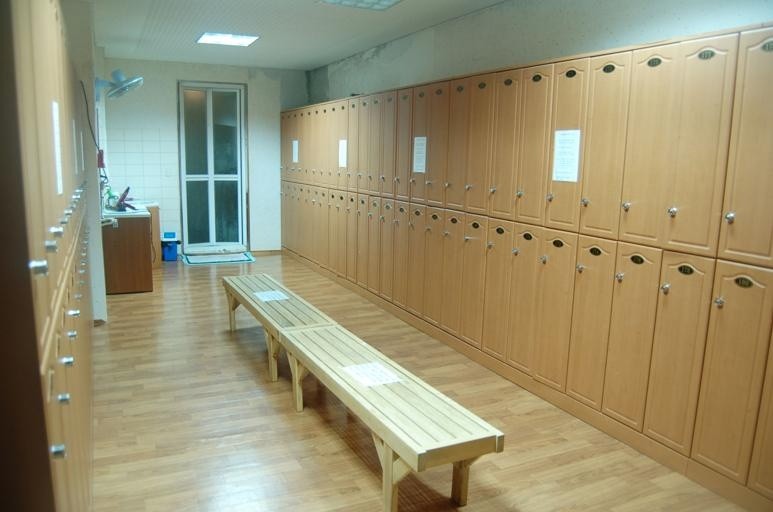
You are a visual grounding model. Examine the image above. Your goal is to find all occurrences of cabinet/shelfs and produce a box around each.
[102,208,161,295]
[602,23,773,512]
[0,1,95,512]
[279,86,408,324]
[407,51,600,429]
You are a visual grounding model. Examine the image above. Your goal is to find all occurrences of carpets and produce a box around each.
[181,252,255,264]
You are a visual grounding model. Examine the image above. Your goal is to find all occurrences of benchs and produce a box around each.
[219,272,335,383]
[279,325,505,511]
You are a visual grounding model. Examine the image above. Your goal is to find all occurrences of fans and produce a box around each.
[96,68,144,103]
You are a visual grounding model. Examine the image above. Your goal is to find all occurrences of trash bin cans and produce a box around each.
[160,231,178,261]
